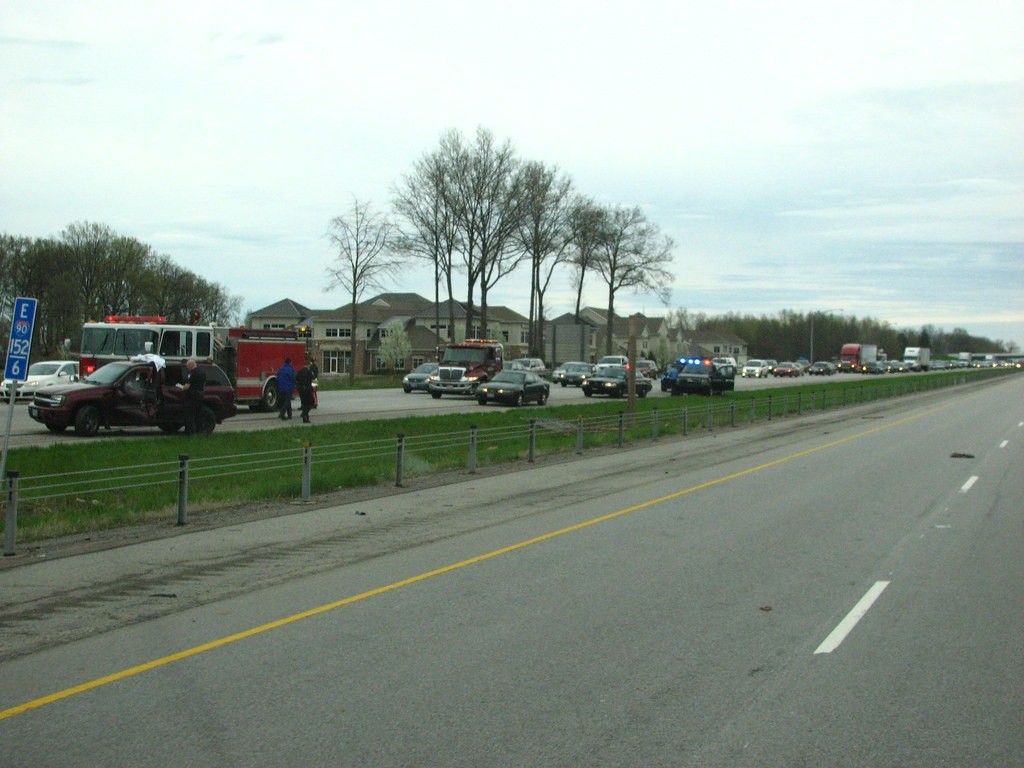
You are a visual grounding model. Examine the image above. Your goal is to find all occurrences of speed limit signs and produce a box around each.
[15,320,29,337]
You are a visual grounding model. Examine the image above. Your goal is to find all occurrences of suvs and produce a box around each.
[28,358,238,436]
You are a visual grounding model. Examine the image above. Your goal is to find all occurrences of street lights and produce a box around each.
[810,308,843,365]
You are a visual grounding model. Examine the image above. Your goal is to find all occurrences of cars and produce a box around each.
[475,369,550,407]
[503,358,546,372]
[551,355,658,399]
[0,361,80,404]
[863,352,1024,374]
[741,359,837,378]
[661,356,737,396]
[402,362,439,394]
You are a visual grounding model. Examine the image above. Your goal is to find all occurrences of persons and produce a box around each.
[668,366,678,396]
[175,360,207,435]
[296,355,318,410]
[137,373,151,389]
[296,360,316,424]
[276,358,297,419]
[674,364,682,374]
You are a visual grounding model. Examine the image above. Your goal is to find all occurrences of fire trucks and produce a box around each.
[79,311,308,412]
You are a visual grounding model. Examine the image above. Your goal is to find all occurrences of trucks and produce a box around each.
[429,338,548,399]
[903,347,931,365]
[836,344,878,372]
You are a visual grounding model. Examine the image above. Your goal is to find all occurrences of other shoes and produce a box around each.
[279,414,286,420]
[301,412,310,423]
[287,415,292,419]
